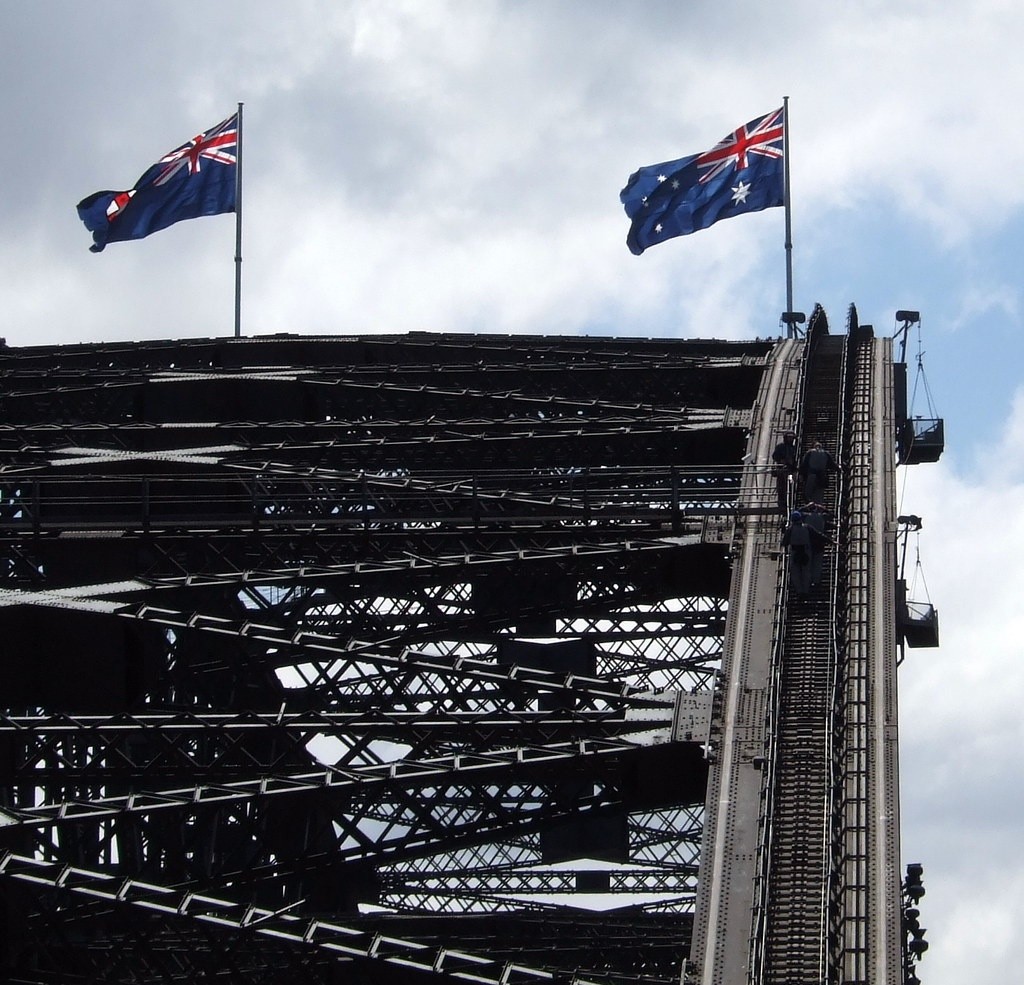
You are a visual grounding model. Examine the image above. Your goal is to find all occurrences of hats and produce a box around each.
[786,430,795,438]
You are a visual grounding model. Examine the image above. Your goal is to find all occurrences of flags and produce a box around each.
[621,108,787,255]
[77,113,241,253]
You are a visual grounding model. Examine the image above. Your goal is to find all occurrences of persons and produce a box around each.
[781,511,841,598]
[797,502,835,586]
[773,430,797,508]
[801,442,840,502]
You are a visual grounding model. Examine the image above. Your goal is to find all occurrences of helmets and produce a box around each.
[791,511,801,521]
